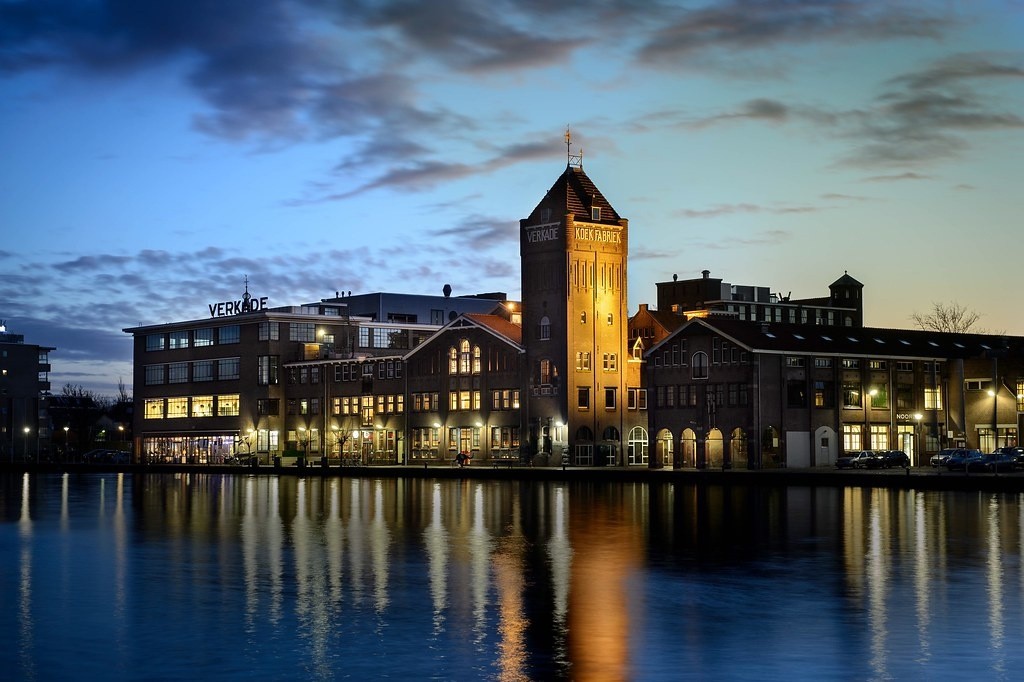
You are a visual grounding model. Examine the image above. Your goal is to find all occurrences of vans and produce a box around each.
[993,446,1024,469]
[929,448,964,469]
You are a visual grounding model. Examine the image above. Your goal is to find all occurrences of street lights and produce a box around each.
[118,425,124,442]
[62,426,70,448]
[21,425,32,463]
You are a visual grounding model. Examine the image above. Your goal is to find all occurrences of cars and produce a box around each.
[944,449,984,471]
[834,450,877,470]
[95,450,130,464]
[866,450,911,470]
[82,449,108,465]
[966,453,1017,473]
